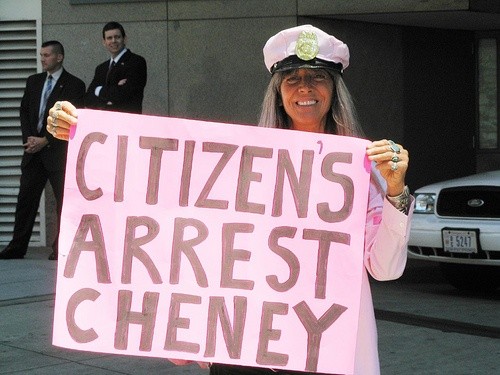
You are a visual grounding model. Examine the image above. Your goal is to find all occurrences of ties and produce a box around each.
[102,61,115,99]
[36,74,53,134]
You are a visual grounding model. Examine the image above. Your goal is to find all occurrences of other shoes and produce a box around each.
[0,246,25,259]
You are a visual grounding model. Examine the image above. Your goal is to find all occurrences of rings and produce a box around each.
[392,152,400,162]
[51,111,60,118]
[51,117,57,126]
[54,101,64,110]
[50,127,57,137]
[391,162,398,171]
[388,140,396,146]
[389,145,401,153]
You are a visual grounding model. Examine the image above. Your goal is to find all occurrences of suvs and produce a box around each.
[407,169,500,292]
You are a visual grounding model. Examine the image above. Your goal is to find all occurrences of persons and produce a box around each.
[86,22,148,115]
[46,23,415,375]
[0,40,86,260]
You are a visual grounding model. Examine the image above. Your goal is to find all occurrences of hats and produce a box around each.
[262,23,350,78]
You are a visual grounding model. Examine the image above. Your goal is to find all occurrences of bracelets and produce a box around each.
[387,184,410,215]
